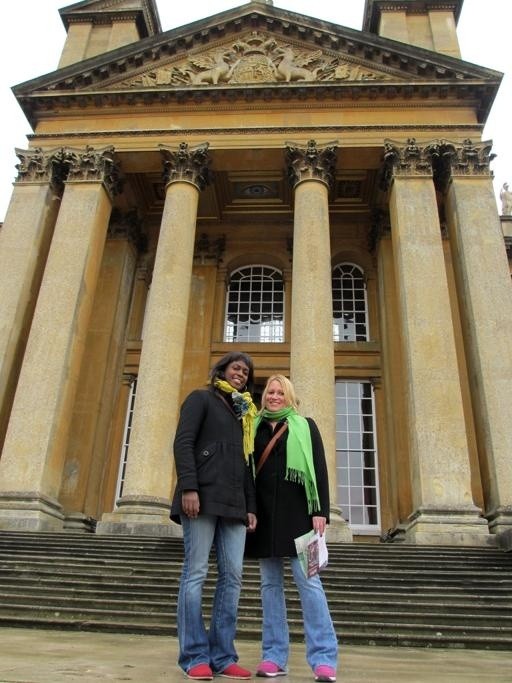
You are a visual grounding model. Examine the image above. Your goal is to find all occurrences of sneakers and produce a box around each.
[312,664,337,681]
[213,660,252,680]
[256,660,288,678]
[185,663,213,680]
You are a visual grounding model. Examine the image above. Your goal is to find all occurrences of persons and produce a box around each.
[243,372,341,682]
[169,351,258,679]
